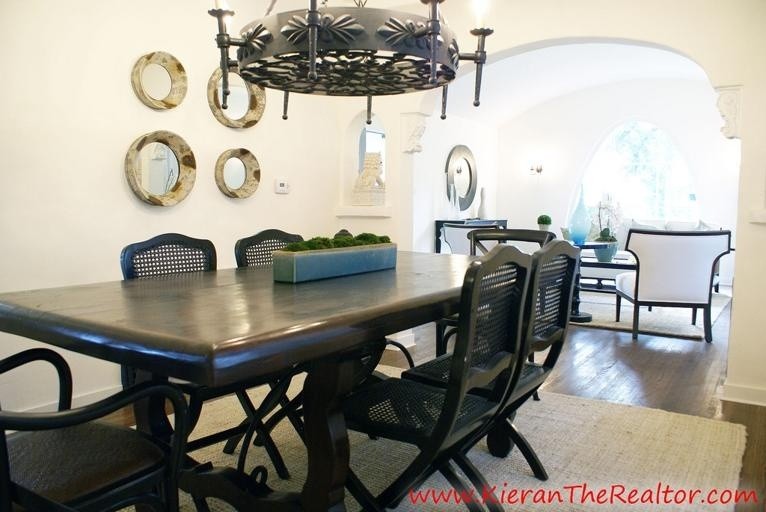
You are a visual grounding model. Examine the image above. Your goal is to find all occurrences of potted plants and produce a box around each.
[537,214,552,232]
[594,226,619,262]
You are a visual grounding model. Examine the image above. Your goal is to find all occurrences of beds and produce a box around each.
[579,248,637,289]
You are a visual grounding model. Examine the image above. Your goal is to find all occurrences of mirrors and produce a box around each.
[125,129,197,207]
[446,144,477,211]
[132,48,188,110]
[208,64,267,129]
[215,147,262,199]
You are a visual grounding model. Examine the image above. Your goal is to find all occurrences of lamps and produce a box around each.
[204,0,496,124]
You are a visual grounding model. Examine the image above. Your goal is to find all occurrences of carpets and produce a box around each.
[569,292,731,340]
[104,360,750,512]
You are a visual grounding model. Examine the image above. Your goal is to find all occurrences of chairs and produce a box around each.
[0,225,582,510]
[436,219,508,258]
[615,228,731,343]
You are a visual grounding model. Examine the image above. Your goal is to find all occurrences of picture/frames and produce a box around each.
[334,108,392,216]
